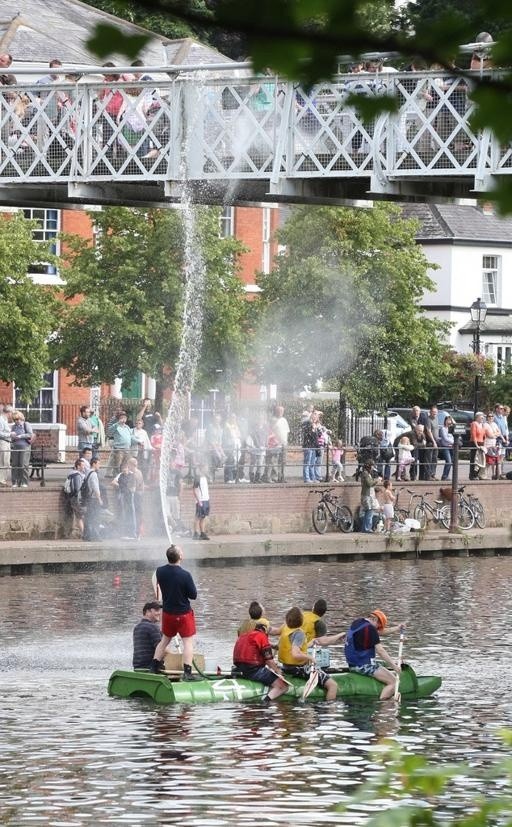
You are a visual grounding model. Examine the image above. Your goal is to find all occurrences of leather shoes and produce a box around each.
[181,671,204,682]
[148,665,168,677]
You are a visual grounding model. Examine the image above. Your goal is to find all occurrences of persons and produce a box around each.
[301,404,344,483]
[150,545,205,681]
[167,461,181,531]
[68,398,166,539]
[128,701,400,820]
[406,57,496,159]
[202,56,402,158]
[232,599,346,702]
[345,610,407,700]
[11,410,33,488]
[352,403,511,533]
[133,602,169,671]
[0,404,14,487]
[2,53,173,158]
[167,402,291,483]
[193,461,213,538]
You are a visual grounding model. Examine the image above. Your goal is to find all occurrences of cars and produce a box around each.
[344,405,490,461]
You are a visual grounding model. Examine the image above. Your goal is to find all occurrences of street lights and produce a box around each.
[468,297,489,417]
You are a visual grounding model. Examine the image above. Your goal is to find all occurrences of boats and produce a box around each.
[104,659,441,707]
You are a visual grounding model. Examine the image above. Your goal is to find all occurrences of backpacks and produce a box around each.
[63,473,80,498]
[80,470,93,498]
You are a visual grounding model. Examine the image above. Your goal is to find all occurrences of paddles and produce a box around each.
[302,644,319,699]
[393,625,402,705]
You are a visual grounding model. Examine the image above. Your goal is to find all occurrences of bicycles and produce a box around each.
[310,484,485,537]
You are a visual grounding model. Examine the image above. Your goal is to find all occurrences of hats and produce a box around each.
[116,410,129,420]
[370,610,387,633]
[143,602,165,610]
[364,458,376,468]
[256,617,270,635]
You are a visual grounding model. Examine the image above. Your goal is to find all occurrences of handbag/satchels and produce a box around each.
[26,433,37,444]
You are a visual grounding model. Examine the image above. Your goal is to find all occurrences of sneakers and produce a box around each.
[426,474,507,481]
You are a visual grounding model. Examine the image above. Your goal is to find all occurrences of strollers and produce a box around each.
[96,482,121,541]
